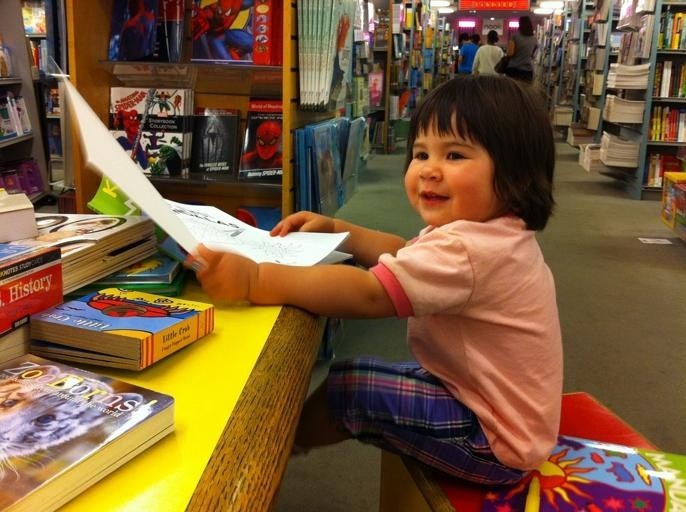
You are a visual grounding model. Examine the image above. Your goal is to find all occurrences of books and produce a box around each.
[27,287,214,371]
[1,190,182,366]
[648,11,686,188]
[51,56,358,273]
[105,1,285,234]
[0,355,174,512]
[535,0,653,173]
[296,1,365,218]
[659,172,686,239]
[354,1,454,158]
[0,3,65,197]
[482,435,685,512]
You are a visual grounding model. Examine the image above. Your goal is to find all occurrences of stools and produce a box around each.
[377,390,685,511]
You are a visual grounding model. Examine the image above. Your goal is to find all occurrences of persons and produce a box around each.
[179,72,567,489]
[458,16,540,86]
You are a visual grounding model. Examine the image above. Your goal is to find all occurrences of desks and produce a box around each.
[0,195,340,512]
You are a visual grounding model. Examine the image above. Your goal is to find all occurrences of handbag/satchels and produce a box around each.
[495,57,509,73]
